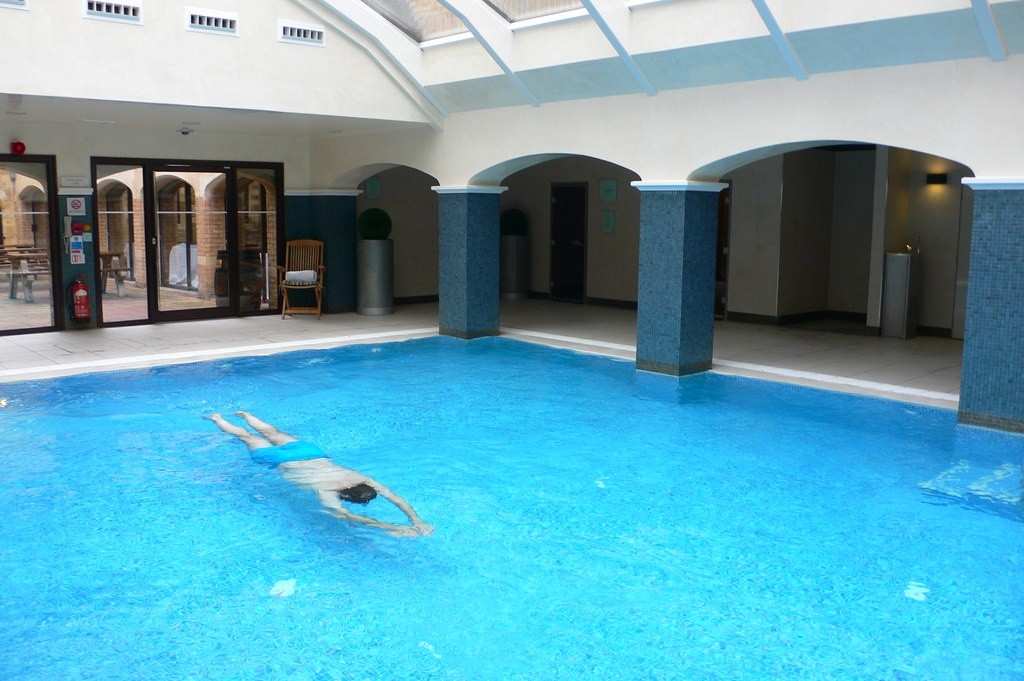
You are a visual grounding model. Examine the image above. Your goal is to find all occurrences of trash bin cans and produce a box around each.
[356,235,394,314]
[499,232,534,301]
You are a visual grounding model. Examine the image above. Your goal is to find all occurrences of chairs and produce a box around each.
[276,240,324,320]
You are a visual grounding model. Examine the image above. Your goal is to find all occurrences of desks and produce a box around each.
[6,251,132,303]
[0,244,33,263]
[0,247,48,280]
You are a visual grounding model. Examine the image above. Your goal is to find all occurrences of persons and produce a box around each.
[202,409,433,538]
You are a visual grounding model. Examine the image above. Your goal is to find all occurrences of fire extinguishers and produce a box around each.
[65,276,91,324]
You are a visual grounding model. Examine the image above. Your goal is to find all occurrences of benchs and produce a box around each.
[0,260,130,275]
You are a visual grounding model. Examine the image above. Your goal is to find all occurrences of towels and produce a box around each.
[286,270,318,281]
